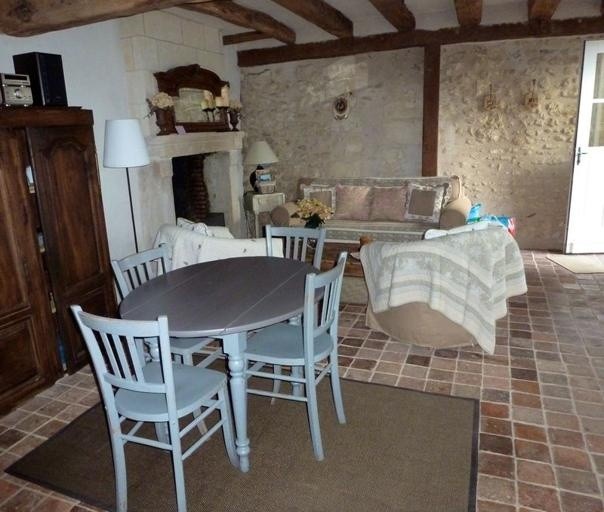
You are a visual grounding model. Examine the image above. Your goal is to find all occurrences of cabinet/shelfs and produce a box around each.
[0,107,119,419]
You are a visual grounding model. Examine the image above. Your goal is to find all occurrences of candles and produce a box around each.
[199,90,214,109]
[215,84,230,107]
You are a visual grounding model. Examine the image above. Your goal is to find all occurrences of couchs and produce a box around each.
[271,175,472,242]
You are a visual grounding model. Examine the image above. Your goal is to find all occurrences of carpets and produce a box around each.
[545,254,604,274]
[3,356,480,512]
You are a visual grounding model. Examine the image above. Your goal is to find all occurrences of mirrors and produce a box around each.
[152,64,231,131]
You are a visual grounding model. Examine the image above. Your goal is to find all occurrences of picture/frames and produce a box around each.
[255,169,277,187]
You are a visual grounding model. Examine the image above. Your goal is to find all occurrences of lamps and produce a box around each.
[103,118,151,253]
[242,139,279,192]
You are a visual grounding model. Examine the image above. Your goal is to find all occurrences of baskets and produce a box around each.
[255,180,277,194]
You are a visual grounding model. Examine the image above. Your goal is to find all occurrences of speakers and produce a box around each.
[12,52,68,108]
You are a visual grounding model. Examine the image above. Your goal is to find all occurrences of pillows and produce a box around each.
[176,217,211,237]
[301,184,450,224]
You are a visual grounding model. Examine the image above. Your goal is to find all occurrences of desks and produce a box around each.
[243,192,285,238]
[118,255,327,474]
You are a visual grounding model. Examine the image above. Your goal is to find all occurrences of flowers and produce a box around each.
[143,91,178,119]
[227,99,242,114]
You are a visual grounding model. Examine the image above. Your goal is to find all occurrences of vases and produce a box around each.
[155,110,177,136]
[229,110,240,131]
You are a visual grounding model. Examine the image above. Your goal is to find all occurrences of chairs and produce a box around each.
[249,225,324,407]
[360,220,528,347]
[109,242,222,441]
[73,307,240,512]
[247,250,348,462]
